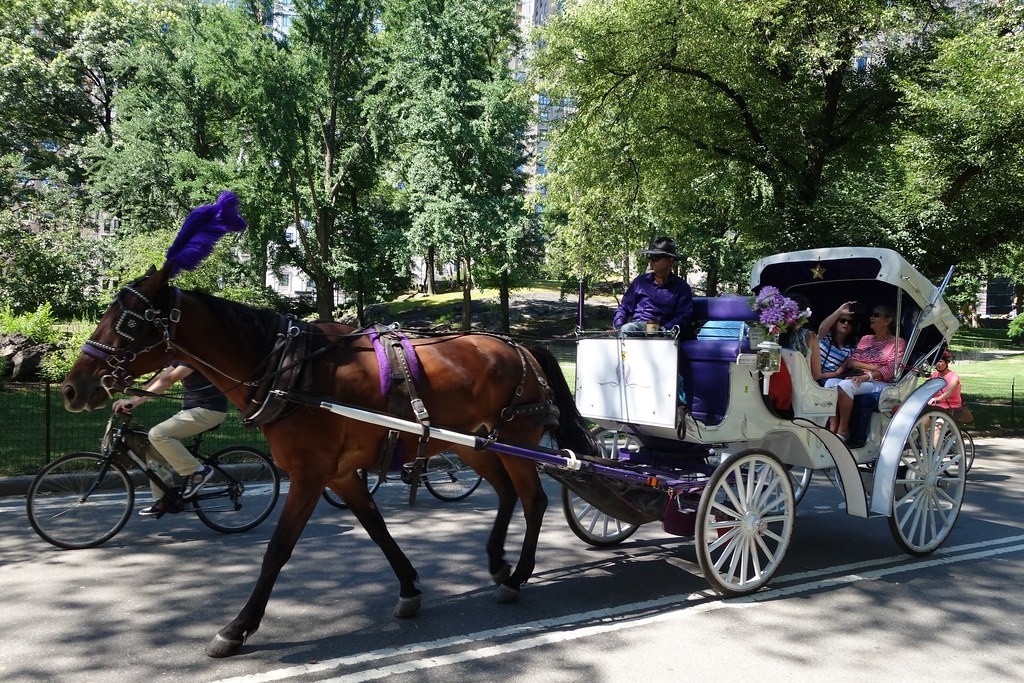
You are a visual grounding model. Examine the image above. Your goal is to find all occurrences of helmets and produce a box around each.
[941,351,953,361]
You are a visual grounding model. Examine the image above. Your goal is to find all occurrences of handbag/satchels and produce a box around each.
[951,404,974,423]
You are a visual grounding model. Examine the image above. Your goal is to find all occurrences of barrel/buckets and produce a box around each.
[714,501,744,549]
[662,492,706,537]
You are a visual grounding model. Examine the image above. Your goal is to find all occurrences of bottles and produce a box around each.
[149,460,171,483]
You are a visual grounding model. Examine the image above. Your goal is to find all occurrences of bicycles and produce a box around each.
[939,429,976,478]
[320,449,484,509]
[25,404,281,550]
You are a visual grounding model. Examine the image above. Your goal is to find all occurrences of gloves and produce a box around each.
[658,326,667,337]
[616,324,624,334]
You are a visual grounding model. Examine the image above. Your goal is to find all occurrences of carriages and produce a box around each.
[63,192,969,657]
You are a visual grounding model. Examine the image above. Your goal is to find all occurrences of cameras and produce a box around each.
[849,302,870,315]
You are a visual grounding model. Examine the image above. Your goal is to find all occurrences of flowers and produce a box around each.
[749,287,813,342]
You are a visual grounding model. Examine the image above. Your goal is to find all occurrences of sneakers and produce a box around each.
[138,498,165,515]
[183,464,215,499]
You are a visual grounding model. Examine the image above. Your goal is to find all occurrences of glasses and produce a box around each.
[870,312,885,317]
[838,318,854,325]
[646,254,665,262]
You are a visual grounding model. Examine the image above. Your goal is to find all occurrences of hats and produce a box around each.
[637,237,684,261]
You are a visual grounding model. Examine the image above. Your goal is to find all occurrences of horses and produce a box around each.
[61,264,599,659]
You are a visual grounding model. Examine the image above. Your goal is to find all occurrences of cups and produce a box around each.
[646,321,659,336]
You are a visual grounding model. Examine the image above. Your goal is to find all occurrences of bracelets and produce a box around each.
[864,372,873,382]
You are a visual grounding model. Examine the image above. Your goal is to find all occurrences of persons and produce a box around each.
[923,351,963,455]
[773,295,906,444]
[613,237,692,338]
[113,359,226,515]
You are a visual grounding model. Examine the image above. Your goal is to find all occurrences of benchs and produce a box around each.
[680,296,762,361]
[758,347,839,417]
[853,349,926,412]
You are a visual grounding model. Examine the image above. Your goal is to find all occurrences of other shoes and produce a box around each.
[837,433,851,443]
